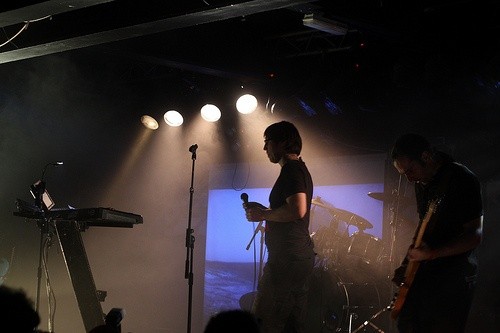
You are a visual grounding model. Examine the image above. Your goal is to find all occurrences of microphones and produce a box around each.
[42,161,63,177]
[240,192,248,203]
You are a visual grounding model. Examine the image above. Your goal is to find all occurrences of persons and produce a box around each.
[392,133,484,333]
[242,121,316,333]
[0,285,50,333]
[203,310,261,333]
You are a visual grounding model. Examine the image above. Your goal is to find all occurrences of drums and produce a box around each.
[311,264,386,333]
[311,225,349,267]
[344,231,387,273]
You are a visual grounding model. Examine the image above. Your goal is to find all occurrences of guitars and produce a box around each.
[390,190,447,318]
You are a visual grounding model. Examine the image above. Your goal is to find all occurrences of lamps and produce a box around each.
[303,13,348,35]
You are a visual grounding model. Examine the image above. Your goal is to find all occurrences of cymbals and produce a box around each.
[312,197,333,210]
[329,207,373,230]
[367,189,415,206]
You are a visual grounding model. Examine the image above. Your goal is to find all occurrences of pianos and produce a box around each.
[13,207,145,333]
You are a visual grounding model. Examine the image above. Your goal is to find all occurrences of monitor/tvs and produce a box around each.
[31,181,55,211]
